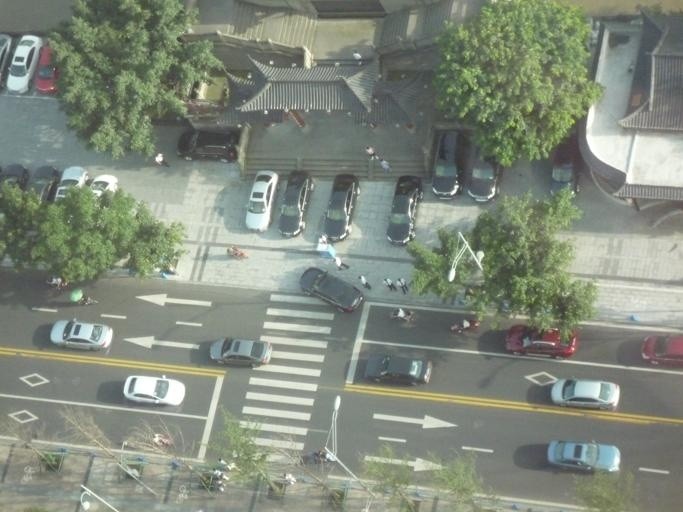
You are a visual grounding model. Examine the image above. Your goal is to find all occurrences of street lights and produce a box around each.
[79,485,120,512]
[116,442,160,497]
[447,232,489,282]
[324,395,377,499]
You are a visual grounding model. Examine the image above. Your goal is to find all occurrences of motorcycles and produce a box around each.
[389,309,417,322]
[450,318,481,334]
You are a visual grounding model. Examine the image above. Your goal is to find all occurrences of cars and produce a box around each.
[548,143,580,197]
[48,318,114,351]
[121,375,186,406]
[317,173,361,243]
[0,164,119,203]
[365,354,432,386]
[641,335,683,366]
[385,176,424,247]
[432,131,502,203]
[0,33,62,94]
[209,337,273,368]
[245,169,279,234]
[277,171,315,239]
[546,439,621,473]
[299,266,364,314]
[176,128,238,163]
[505,324,578,361]
[551,378,620,411]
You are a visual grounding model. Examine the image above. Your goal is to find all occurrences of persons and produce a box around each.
[331,257,348,271]
[379,158,391,172]
[365,146,379,161]
[383,278,396,291]
[357,276,370,290]
[45,276,67,297]
[311,449,336,464]
[318,235,327,245]
[283,473,296,484]
[226,246,242,260]
[210,457,241,495]
[76,295,87,307]
[153,153,169,168]
[352,50,362,67]
[396,278,408,295]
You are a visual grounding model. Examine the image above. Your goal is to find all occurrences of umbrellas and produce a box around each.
[67,288,81,302]
